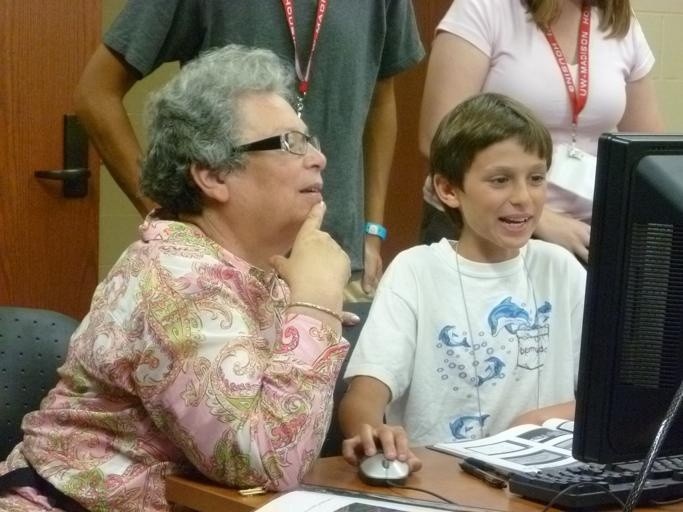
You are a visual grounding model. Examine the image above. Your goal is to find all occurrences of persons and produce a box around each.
[412,0,668,282]
[0,41,363,512]
[329,90,591,473]
[68,1,431,459]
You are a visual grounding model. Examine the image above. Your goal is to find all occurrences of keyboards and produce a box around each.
[508,456,683,512]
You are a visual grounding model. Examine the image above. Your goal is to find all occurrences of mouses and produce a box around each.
[356,449,410,485]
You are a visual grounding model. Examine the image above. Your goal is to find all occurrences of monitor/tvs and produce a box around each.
[570,132,683,465]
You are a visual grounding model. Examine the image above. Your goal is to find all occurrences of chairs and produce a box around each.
[321,302,373,458]
[0,306,81,464]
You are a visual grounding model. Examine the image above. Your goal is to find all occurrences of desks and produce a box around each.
[165,446,683,512]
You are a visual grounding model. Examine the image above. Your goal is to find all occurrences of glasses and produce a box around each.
[232,132,322,155]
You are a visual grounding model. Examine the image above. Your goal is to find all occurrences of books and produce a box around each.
[426,416,582,472]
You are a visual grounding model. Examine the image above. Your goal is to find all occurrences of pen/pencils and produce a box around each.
[458,458,509,489]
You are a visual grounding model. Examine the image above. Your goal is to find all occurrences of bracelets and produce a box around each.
[285,300,344,325]
[364,221,388,242]
